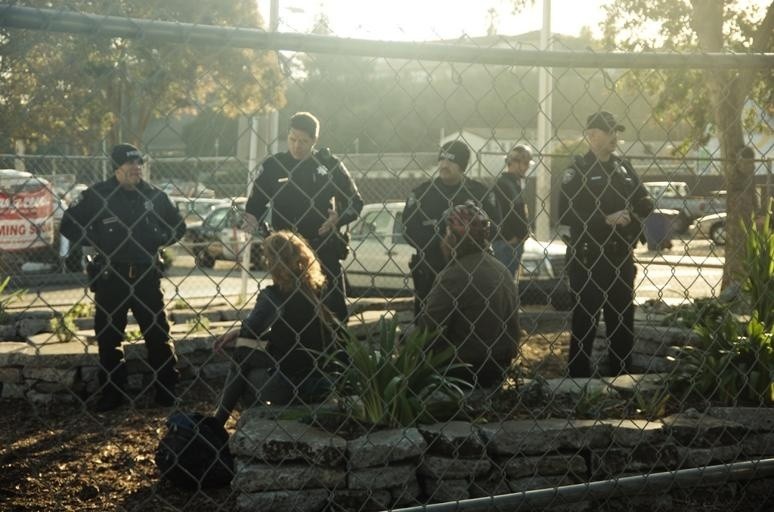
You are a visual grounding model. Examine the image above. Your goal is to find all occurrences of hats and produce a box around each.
[112,143,144,173]
[587,111,626,135]
[437,140,471,170]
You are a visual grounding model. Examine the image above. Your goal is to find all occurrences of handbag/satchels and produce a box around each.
[156,413,233,491]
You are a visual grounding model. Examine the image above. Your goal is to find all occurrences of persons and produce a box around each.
[194,231,339,430]
[417,201,522,391]
[59,142,186,413]
[557,110,655,380]
[243,112,363,296]
[489,143,533,337]
[402,138,500,322]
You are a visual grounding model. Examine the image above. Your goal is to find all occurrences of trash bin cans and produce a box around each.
[647,208,679,252]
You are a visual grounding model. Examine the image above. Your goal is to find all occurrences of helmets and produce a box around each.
[442,202,491,238]
[509,144,536,166]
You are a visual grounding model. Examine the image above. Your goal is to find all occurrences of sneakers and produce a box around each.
[154,389,176,406]
[95,392,123,409]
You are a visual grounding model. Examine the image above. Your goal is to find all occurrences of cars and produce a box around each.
[341,201,571,312]
[184,197,272,270]
[1,164,215,274]
[688,212,726,244]
